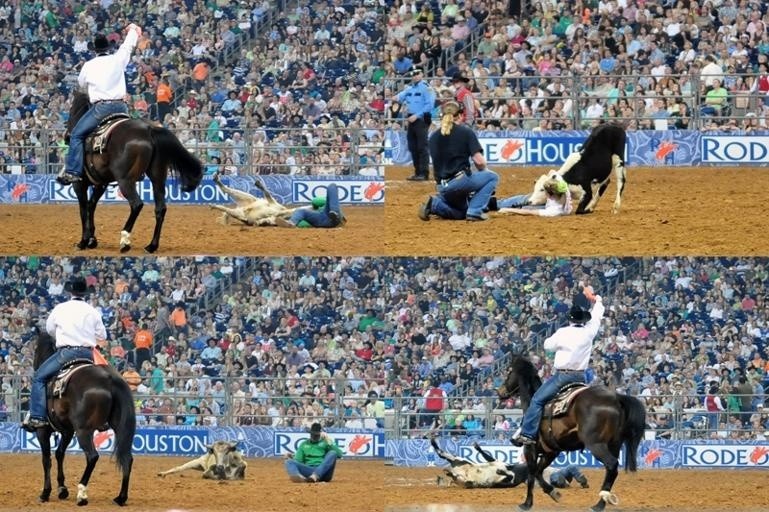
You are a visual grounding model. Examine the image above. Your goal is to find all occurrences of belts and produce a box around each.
[96,99,122,103]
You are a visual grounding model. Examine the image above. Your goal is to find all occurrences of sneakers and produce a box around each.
[61,173,82,182]
[466,214,487,221]
[20,418,46,428]
[328,211,340,224]
[418,195,432,221]
[307,473,318,483]
[276,216,295,228]
[559,476,566,488]
[290,475,306,482]
[577,476,587,486]
[517,435,536,445]
[406,174,428,180]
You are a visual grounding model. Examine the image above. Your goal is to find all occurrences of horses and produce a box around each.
[497,346,646,512]
[63,88,202,254]
[33,332,136,505]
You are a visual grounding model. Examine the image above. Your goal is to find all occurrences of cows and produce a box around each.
[208,172,313,226]
[527,122,626,214]
[157,439,247,479]
[431,437,529,488]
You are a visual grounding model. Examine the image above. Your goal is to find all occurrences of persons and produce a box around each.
[276,183,346,227]
[285,423,343,482]
[1,0,755,184]
[521,454,589,489]
[469,169,573,218]
[0,256,769,445]
[419,103,499,222]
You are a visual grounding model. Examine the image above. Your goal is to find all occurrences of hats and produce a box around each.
[64,276,90,295]
[308,423,321,435]
[212,156,221,164]
[408,66,424,76]
[566,304,591,323]
[447,72,470,84]
[87,34,116,51]
